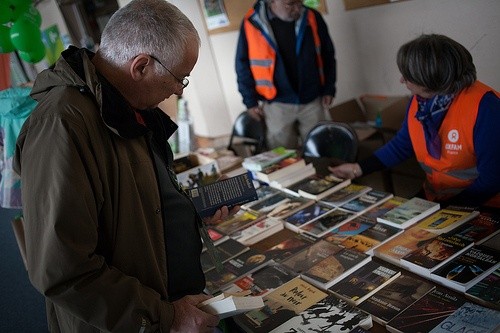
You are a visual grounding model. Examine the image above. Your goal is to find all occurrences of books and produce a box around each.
[175,147,500,333]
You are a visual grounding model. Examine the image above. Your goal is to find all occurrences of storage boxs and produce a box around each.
[327,94,417,197]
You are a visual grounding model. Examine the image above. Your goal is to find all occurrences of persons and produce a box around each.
[11,0,218,333]
[329,33,500,208]
[234,0,337,151]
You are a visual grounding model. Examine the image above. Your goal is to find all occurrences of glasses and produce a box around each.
[133,53,189,90]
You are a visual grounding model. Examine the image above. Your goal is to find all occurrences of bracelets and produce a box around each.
[349,163,357,178]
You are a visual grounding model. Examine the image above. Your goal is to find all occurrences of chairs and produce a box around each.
[299,120,362,170]
[226,110,267,157]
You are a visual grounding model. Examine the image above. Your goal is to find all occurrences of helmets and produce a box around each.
[228,110,267,154]
[302,122,357,163]
